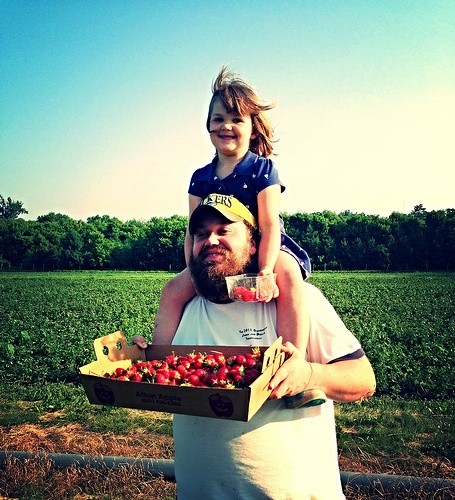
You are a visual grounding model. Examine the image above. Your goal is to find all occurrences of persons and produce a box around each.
[151,63,327,410]
[131,193,376,500]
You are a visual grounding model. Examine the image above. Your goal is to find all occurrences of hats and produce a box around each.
[187,192,257,235]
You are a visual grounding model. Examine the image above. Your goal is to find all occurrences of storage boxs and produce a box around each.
[79,331,286,423]
[225,273,277,302]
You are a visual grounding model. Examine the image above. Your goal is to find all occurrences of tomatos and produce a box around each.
[230,285,270,303]
[102,351,265,389]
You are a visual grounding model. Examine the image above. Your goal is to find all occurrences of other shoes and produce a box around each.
[284,389,327,410]
